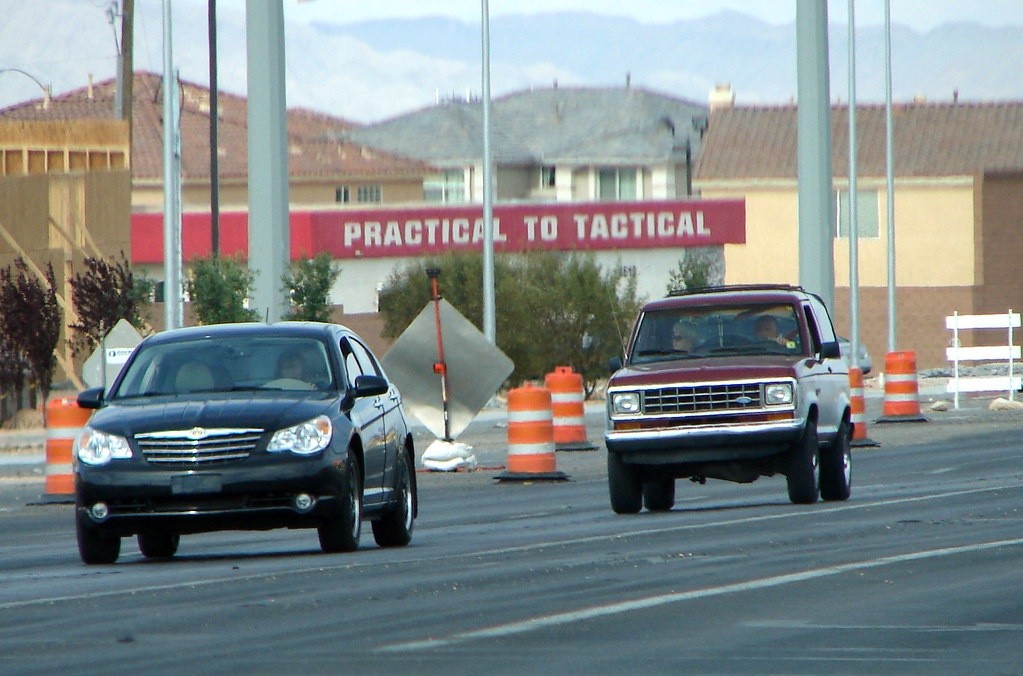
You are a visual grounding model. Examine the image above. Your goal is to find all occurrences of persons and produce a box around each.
[277,349,329,390]
[672,321,699,351]
[754,315,796,345]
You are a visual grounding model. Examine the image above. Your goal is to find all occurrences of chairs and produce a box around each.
[745,315,799,350]
[173,359,214,394]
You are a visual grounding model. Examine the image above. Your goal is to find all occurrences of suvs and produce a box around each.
[604,283,854,516]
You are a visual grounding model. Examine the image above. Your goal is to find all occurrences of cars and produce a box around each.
[696,313,870,379]
[71,320,417,565]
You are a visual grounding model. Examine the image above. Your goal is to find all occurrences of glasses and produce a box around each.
[671,335,686,342]
